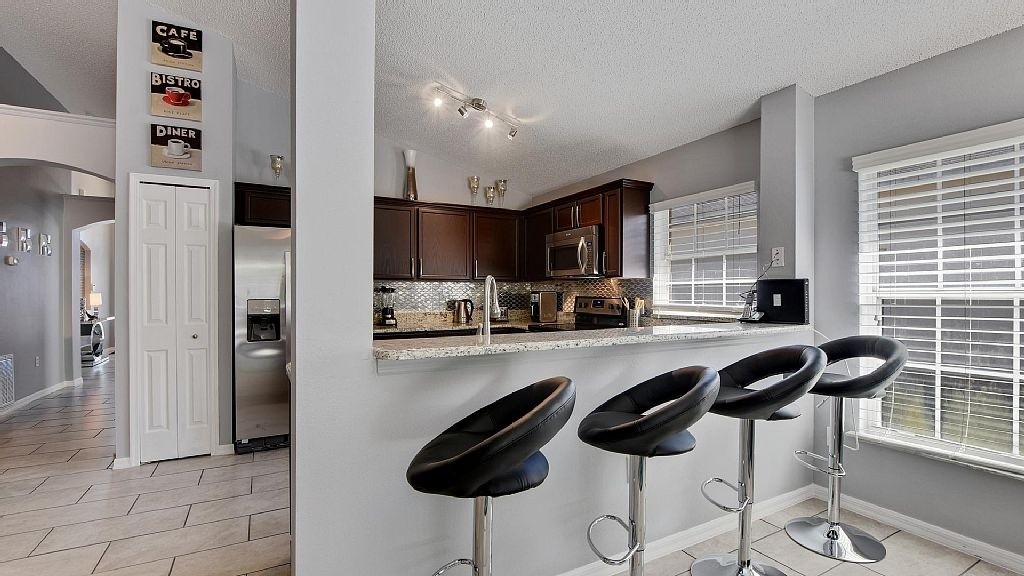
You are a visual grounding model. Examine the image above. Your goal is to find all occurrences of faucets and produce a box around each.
[480,336,490,345]
[476,274,501,336]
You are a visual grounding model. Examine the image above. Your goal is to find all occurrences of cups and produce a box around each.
[166,87,191,102]
[168,139,190,155]
[161,38,187,53]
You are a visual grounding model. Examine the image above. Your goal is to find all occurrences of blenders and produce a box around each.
[381,288,397,327]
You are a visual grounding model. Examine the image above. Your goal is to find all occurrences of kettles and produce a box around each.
[452,299,473,323]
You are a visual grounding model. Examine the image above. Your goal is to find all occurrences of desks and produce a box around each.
[80,317,115,367]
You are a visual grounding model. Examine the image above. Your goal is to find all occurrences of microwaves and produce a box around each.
[546,225,605,278]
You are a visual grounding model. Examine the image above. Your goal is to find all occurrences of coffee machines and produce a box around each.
[528,290,560,323]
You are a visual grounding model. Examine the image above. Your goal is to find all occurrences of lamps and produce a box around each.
[90,293,102,315]
[434,87,519,140]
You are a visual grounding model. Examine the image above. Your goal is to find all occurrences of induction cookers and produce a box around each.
[528,296,627,332]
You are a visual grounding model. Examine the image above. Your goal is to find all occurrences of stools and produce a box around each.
[406,376,575,576]
[784,335,909,563]
[578,366,720,576]
[691,345,828,576]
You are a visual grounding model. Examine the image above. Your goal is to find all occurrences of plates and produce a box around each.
[162,48,192,59]
[162,149,191,159]
[163,95,191,106]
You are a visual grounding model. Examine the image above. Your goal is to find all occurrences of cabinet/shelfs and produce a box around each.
[235,182,291,228]
[374,179,654,282]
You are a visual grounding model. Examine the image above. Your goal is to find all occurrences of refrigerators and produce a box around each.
[234,225,291,444]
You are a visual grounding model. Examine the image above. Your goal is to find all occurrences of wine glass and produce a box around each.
[470,175,480,205]
[496,180,507,208]
[486,186,496,206]
